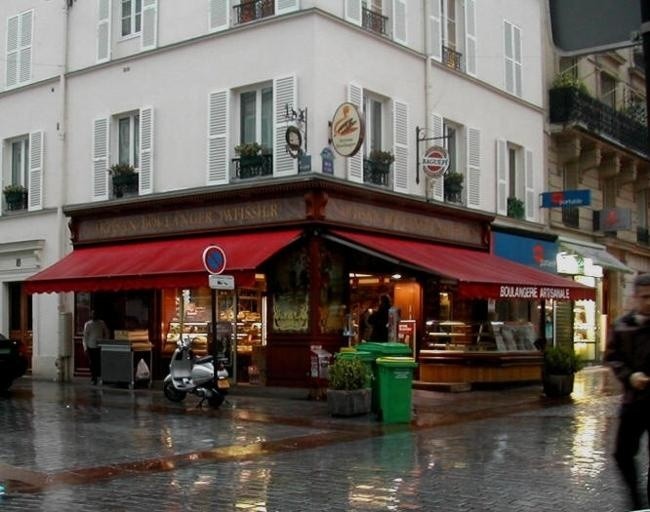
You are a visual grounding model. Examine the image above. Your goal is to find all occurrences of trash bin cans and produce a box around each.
[95,339,155,390]
[335,342,419,425]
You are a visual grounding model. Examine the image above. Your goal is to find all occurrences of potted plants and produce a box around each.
[541,344,586,398]
[234,142,262,167]
[324,359,373,417]
[2,184,24,203]
[370,148,394,174]
[108,162,135,186]
[444,171,464,193]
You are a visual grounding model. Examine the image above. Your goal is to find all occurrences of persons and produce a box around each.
[604,273,650,511]
[367,293,394,341]
[83,307,111,385]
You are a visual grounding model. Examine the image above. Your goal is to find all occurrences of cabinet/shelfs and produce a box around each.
[419,320,543,384]
[163,320,262,382]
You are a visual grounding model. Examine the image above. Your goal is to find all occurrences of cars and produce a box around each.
[0,334,29,393]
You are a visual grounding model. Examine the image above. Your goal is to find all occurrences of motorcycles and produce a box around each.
[163,335,233,409]
